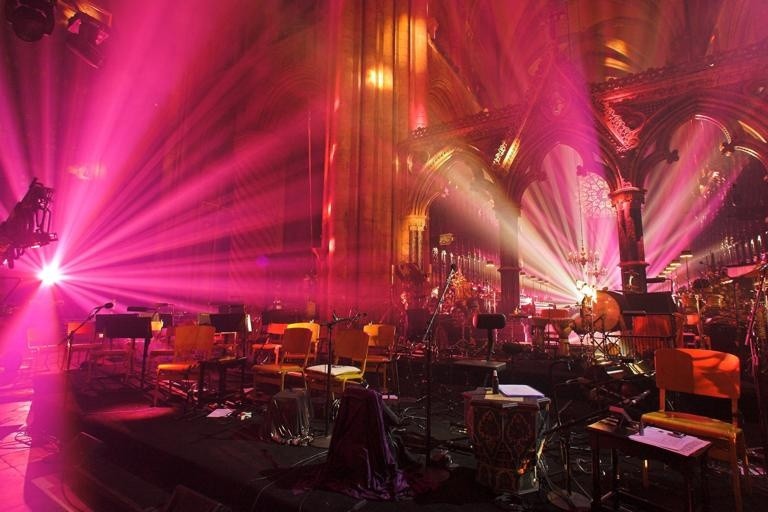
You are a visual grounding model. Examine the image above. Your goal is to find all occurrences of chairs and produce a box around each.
[304,329,370,416]
[250,327,313,394]
[251,323,286,364]
[21,313,247,420]
[354,324,395,390]
[638,346,751,512]
[287,322,319,361]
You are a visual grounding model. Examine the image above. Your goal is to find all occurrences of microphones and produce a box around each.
[94,303,113,309]
[445,264,456,283]
[351,312,368,321]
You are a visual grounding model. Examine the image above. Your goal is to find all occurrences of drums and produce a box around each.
[581,291,631,333]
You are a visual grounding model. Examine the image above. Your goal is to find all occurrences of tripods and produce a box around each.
[540,442,593,502]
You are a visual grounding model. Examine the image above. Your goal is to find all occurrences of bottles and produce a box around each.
[492,370,500,394]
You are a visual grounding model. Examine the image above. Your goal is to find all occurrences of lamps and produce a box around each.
[584,264,609,280]
[63,11,106,70]
[566,148,600,271]
